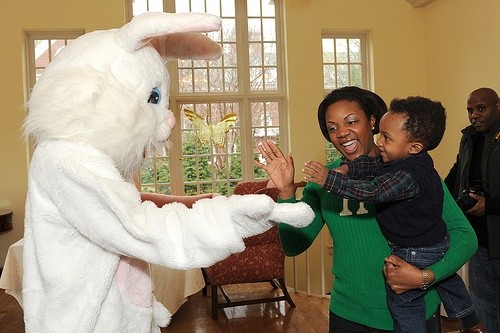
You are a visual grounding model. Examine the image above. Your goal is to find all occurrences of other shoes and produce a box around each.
[459,322,487,333]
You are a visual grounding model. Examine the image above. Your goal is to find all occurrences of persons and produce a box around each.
[302,95,486,333]
[444,87,500,332]
[253,85,478,333]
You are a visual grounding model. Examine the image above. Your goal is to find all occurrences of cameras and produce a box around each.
[456,185,484,212]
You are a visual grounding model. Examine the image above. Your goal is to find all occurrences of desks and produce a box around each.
[0,236,206,326]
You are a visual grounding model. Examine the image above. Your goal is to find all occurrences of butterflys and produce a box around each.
[184,109,237,147]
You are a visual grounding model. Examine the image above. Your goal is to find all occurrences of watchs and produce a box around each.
[420,270,430,290]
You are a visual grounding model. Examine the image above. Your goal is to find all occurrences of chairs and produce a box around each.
[202,179,307,320]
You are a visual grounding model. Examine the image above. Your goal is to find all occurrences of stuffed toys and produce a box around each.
[18,10,315,333]
